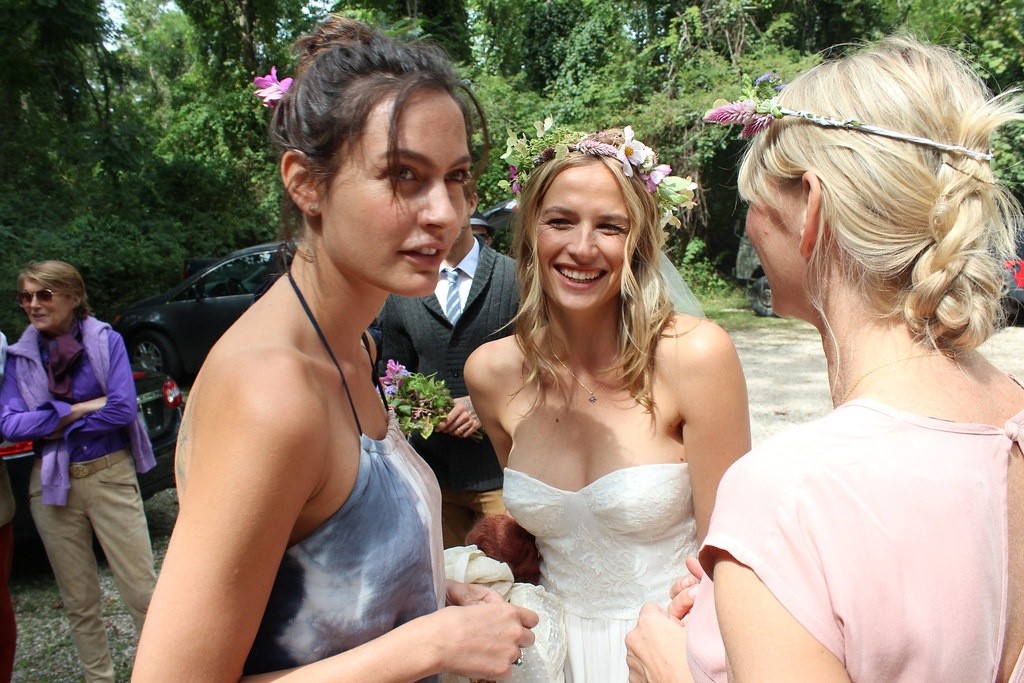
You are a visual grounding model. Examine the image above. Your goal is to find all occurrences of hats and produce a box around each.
[470,211,496,237]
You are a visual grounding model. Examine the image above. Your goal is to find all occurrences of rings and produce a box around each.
[513,648,523,666]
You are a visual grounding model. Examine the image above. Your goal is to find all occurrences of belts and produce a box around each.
[33,447,130,478]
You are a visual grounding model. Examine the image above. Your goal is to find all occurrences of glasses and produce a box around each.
[16,289,61,308]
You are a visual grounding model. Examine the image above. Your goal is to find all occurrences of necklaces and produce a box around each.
[842,350,953,404]
[547,327,619,403]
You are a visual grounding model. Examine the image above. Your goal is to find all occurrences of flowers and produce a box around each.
[376,358,486,446]
[496,111,696,237]
[702,70,864,140]
[248,66,293,111]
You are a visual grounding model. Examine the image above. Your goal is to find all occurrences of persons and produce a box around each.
[444,129,751,683]
[130,13,538,683]
[0,261,157,683]
[625,36,1024,683]
[368,183,520,551]
[470,211,496,246]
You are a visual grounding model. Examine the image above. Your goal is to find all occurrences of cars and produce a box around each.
[2,363,186,555]
[109,240,382,379]
[732,227,1024,330]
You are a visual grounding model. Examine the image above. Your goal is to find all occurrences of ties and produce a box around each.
[440,267,461,326]
